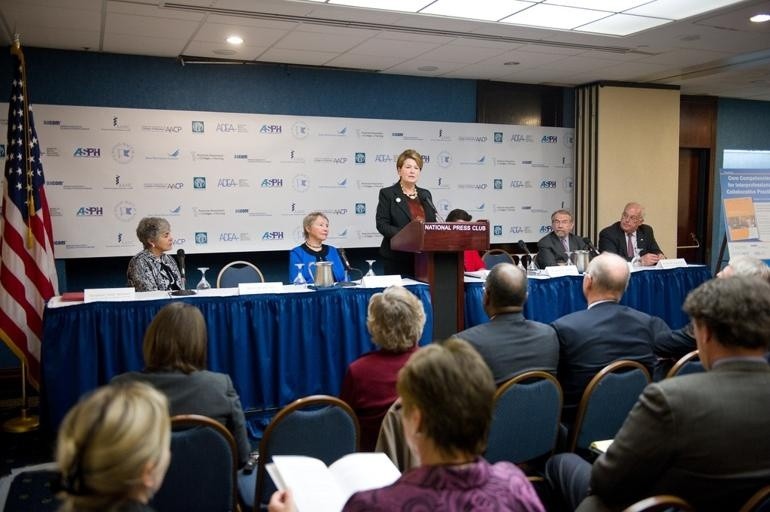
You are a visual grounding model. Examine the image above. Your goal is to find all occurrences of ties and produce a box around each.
[627,233,634,258]
[562,239,567,252]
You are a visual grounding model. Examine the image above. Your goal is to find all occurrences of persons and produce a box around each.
[541,251,770,512]
[376,149,436,274]
[268,338,546,512]
[54,379,172,512]
[112,301,251,470]
[290,212,350,285]
[446,208,487,271]
[535,209,594,269]
[451,261,559,388]
[127,218,184,293]
[339,286,427,452]
[599,204,667,267]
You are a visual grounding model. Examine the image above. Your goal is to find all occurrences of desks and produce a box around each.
[39,272,433,443]
[463,262,709,330]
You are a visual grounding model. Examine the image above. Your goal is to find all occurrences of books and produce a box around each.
[266,453,402,512]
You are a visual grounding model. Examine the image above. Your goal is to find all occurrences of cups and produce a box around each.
[574,249,590,273]
[308,261,336,290]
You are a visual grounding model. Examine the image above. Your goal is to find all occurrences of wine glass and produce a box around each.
[631,249,642,268]
[563,251,573,265]
[197,268,210,293]
[527,254,539,276]
[294,264,306,287]
[515,254,526,274]
[365,260,376,277]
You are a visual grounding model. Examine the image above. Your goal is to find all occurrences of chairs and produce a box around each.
[624,494,699,512]
[237,394,361,511]
[218,260,264,288]
[147,413,237,512]
[666,348,711,376]
[742,486,769,511]
[568,358,652,459]
[483,371,564,465]
[483,248,515,269]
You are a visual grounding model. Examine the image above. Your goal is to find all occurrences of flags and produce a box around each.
[0,34,59,432]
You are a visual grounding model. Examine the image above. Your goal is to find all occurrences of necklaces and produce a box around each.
[402,185,417,197]
[306,241,322,248]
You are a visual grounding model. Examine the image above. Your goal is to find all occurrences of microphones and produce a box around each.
[518,240,530,254]
[582,237,599,257]
[422,191,437,213]
[338,248,351,270]
[177,249,186,277]
